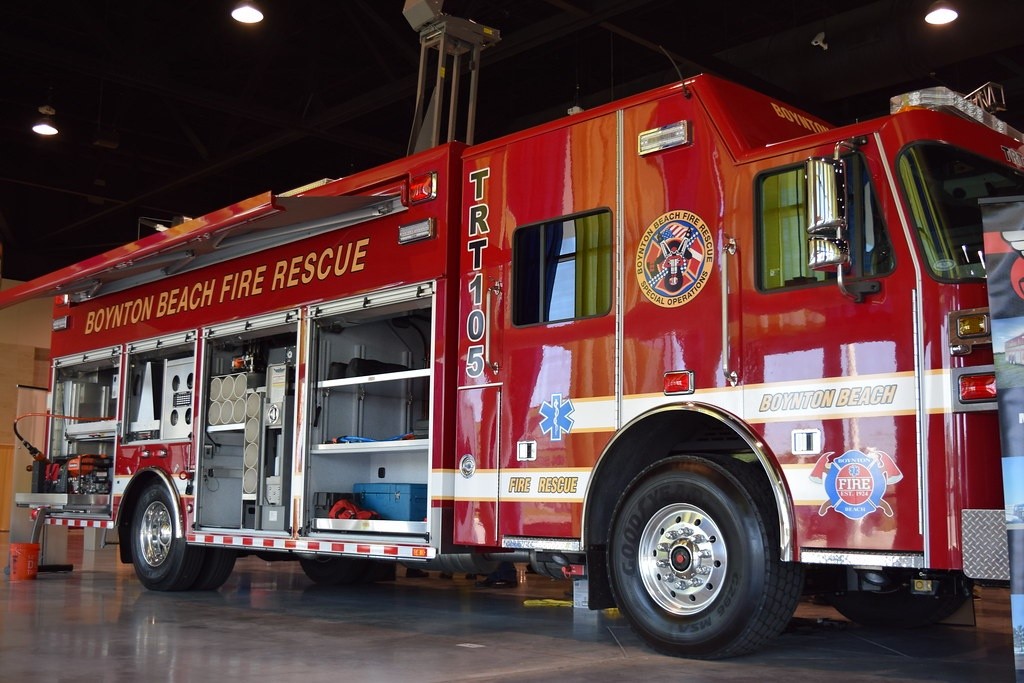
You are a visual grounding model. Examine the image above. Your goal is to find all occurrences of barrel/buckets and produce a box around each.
[10,543,40,580]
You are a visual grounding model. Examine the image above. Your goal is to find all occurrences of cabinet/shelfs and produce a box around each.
[311,292,428,539]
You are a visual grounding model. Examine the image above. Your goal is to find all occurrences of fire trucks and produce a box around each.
[14,72,1023,661]
